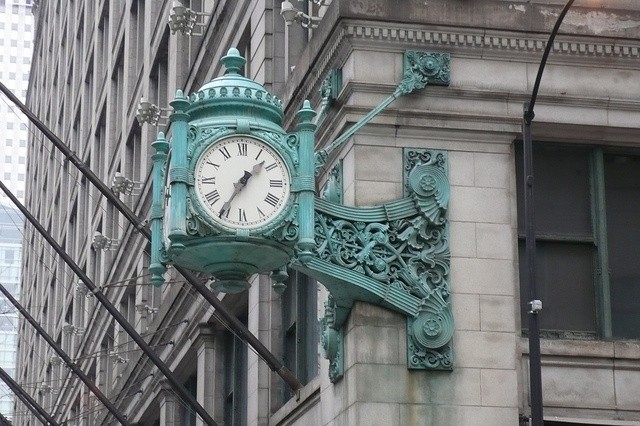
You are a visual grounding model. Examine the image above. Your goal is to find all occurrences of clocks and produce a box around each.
[193,133,295,235]
